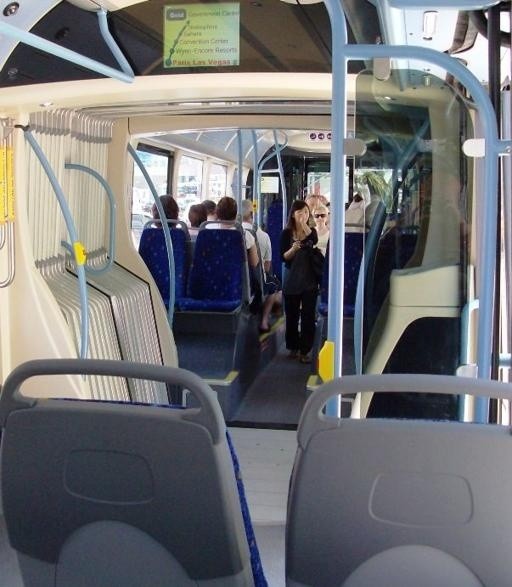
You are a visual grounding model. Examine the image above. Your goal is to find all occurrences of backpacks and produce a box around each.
[282,245,324,294]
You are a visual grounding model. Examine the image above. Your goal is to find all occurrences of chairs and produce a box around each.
[285,374,510,586]
[0,359,269,586]
[138,198,421,319]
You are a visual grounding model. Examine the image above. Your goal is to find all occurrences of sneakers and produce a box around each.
[287,350,310,363]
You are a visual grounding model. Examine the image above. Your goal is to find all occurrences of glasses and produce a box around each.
[315,214,326,218]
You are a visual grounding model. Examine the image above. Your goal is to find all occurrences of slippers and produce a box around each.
[259,323,271,333]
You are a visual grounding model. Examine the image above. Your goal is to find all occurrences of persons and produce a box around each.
[187,204,207,241]
[203,198,218,222]
[279,200,318,363]
[204,197,278,333]
[304,195,331,324]
[237,199,283,305]
[152,195,179,230]
